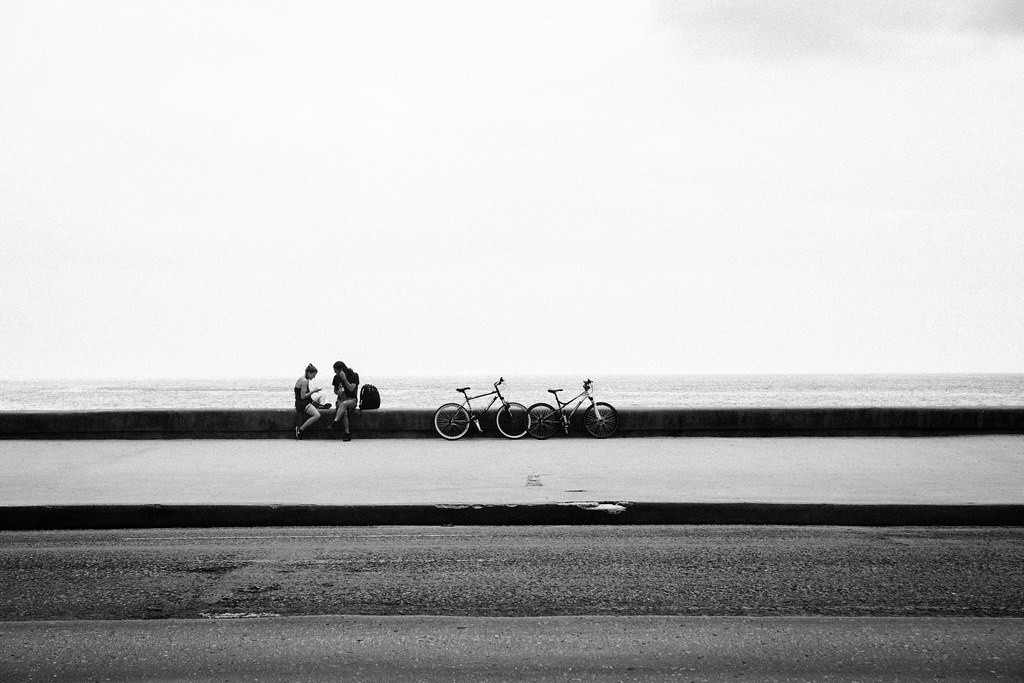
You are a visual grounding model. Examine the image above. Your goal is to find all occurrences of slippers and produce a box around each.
[296,426,303,440]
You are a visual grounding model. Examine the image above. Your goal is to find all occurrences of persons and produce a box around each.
[327,361,360,441]
[294,363,332,440]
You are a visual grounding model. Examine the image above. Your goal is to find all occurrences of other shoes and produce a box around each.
[328,421,337,432]
[343,433,351,441]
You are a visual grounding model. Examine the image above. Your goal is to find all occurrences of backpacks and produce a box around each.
[359,384,380,410]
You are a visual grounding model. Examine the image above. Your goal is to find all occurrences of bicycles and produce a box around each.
[433,375,532,439]
[523,378,620,439]
